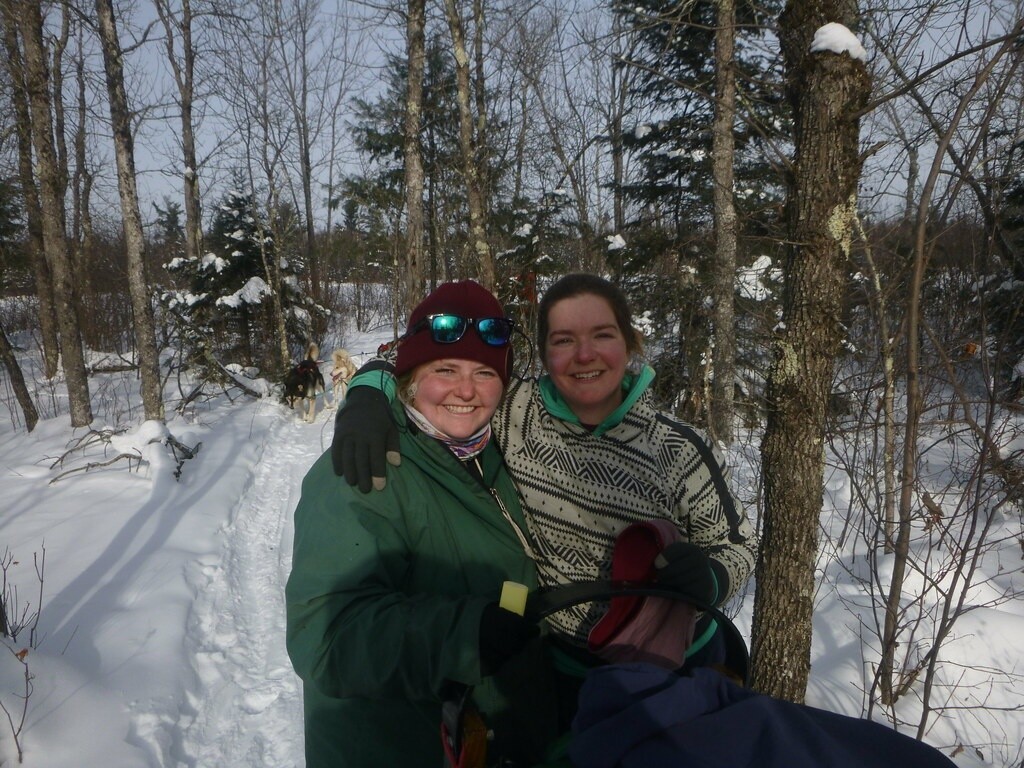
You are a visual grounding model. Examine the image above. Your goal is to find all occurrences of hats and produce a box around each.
[395,280,514,388]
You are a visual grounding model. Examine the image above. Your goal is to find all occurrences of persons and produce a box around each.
[333,275,760,674]
[286,279,545,768]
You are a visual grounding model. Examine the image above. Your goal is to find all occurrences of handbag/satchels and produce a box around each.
[441,580,750,768]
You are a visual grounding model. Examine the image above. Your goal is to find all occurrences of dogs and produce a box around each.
[330,349,358,406]
[282,343,333,423]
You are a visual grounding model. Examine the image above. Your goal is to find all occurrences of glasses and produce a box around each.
[400,312,515,348]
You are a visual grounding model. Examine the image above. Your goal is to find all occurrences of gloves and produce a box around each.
[480,586,545,672]
[331,385,402,494]
[654,541,729,612]
